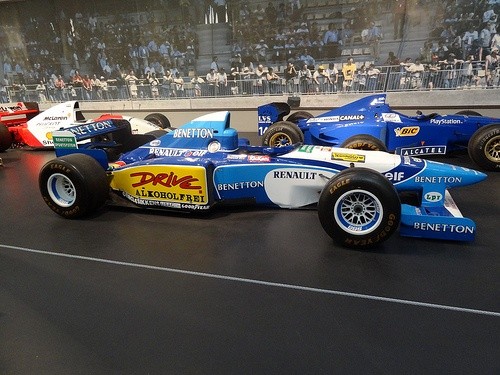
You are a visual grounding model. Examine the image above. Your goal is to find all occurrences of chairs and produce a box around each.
[252,0,374,76]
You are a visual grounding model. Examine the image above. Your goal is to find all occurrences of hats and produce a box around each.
[259,40,264,43]
[187,46,191,49]
[301,23,307,27]
[243,67,249,71]
[432,55,438,59]
[449,53,456,57]
[319,66,326,70]
[230,67,235,71]
[368,64,374,67]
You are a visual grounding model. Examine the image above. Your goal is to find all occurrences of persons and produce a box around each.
[1,0,499,102]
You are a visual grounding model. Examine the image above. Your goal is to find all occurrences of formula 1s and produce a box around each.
[0,101,176,153]
[38,112,490,249]
[255,95,500,172]
